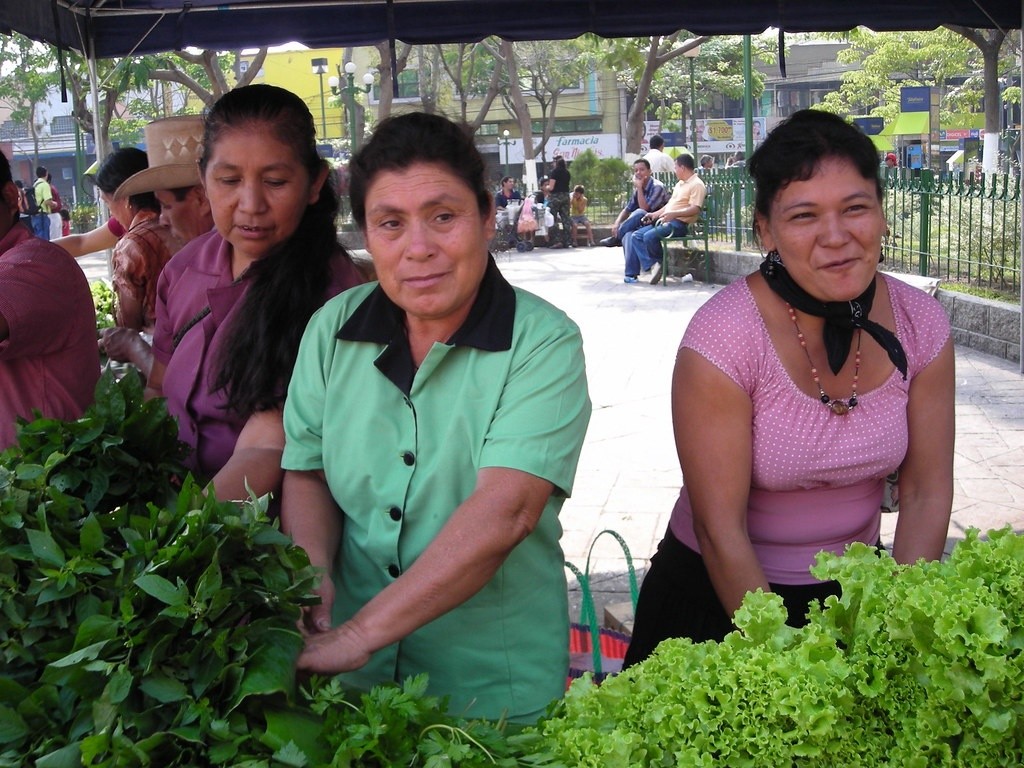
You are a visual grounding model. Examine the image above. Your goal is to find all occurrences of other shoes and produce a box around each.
[500,235,597,252]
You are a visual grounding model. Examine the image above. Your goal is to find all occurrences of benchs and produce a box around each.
[655,194,712,286]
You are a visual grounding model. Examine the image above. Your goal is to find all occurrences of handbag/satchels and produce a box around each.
[565,530,638,697]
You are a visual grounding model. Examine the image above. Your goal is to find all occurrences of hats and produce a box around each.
[111,114,207,202]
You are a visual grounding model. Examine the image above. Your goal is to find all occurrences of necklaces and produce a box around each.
[786,302,861,415]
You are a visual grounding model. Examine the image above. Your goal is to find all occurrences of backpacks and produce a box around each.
[17,182,45,216]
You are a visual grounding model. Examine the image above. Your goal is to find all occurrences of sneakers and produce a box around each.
[624,274,640,283]
[649,261,663,286]
[600,236,622,247]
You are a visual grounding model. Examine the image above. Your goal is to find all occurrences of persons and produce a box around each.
[15,166,70,240]
[0,150,101,457]
[632,154,708,285]
[600,159,668,283]
[540,156,572,248]
[698,154,715,234]
[686,121,707,141]
[281,111,593,725]
[882,152,897,168]
[753,121,764,140]
[643,134,675,180]
[570,184,596,247]
[725,150,746,207]
[495,176,521,209]
[143,84,369,502]
[641,123,649,143]
[620,109,956,670]
[97,114,214,380]
[50,147,180,334]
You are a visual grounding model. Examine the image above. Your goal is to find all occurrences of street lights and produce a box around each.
[311,58,330,143]
[683,39,701,169]
[327,62,374,153]
[496,129,517,177]
